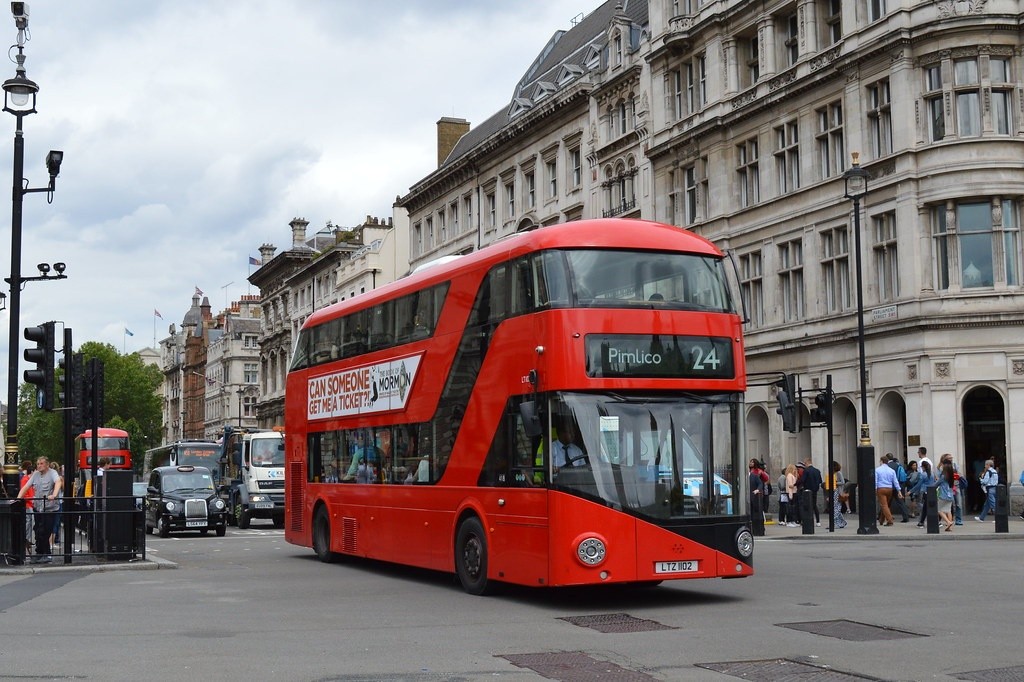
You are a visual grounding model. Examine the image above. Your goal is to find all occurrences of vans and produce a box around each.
[513,414,734,516]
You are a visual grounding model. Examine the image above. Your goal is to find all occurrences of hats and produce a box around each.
[795,461,806,469]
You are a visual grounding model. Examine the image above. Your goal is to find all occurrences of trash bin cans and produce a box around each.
[0,499,27,565]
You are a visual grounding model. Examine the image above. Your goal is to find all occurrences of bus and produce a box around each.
[143,439,221,482]
[284,217,803,596]
[74,426,132,497]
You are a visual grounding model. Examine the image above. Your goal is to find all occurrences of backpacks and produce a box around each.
[896,464,907,482]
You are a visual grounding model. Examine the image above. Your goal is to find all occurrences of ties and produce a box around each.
[562,446,574,467]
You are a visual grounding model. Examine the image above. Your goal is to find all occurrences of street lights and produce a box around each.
[842,151,879,534]
[0,0,68,495]
[236,389,244,425]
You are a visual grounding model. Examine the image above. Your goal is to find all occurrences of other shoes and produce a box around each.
[974,517,984,523]
[880,523,883,525]
[916,523,924,529]
[901,519,908,522]
[816,523,820,526]
[945,521,953,531]
[778,520,801,527]
[884,523,893,526]
[943,526,952,531]
[847,509,851,514]
[939,523,943,527]
[955,523,963,525]
[992,521,995,523]
[1019,513,1024,520]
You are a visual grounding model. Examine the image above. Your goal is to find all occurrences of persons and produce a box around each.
[97,458,110,476]
[257,441,273,462]
[906,447,935,528]
[331,429,429,481]
[778,458,822,527]
[933,454,964,531]
[17,456,64,563]
[1020,470,1024,520]
[875,453,909,526]
[648,294,664,301]
[833,461,851,528]
[331,338,341,359]
[749,459,773,522]
[975,456,999,523]
[552,421,587,478]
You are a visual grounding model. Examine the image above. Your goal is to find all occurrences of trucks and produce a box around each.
[215,426,285,529]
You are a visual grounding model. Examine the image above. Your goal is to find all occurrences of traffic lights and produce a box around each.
[810,393,827,422]
[56,351,84,434]
[23,323,54,411]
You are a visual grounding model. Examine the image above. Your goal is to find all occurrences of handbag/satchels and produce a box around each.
[763,482,772,496]
[958,477,968,490]
[781,491,788,502]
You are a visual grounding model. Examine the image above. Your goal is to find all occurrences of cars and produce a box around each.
[133,465,228,538]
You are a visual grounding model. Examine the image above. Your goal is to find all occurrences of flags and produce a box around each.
[125,329,133,336]
[249,257,262,265]
[196,288,204,297]
[155,310,163,321]
